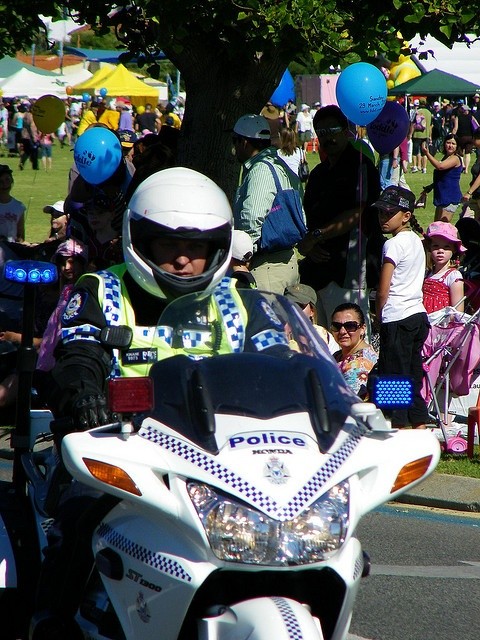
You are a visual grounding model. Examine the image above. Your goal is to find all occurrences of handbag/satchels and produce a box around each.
[413,111,427,132]
[250,160,309,253]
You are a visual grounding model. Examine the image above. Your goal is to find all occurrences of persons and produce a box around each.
[0,163,25,244]
[64,174,134,242]
[376,187,431,429]
[302,105,381,344]
[459,93,472,156]
[283,284,341,356]
[86,203,124,267]
[412,97,432,172]
[43,201,68,234]
[0,100,185,171]
[471,93,480,150]
[431,96,458,152]
[399,135,407,187]
[423,221,464,319]
[261,101,323,150]
[409,100,414,109]
[34,166,289,640]
[378,145,399,191]
[232,115,308,296]
[413,99,420,109]
[35,238,88,372]
[463,127,480,202]
[277,127,308,183]
[421,134,464,220]
[330,303,379,395]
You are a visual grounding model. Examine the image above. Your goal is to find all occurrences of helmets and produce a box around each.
[123,167,235,304]
[422,221,468,253]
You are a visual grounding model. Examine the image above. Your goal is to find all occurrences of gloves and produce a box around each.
[74,392,119,430]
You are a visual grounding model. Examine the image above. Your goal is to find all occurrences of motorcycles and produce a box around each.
[0,289,441,640]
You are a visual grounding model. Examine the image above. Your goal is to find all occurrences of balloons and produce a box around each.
[32,95,66,135]
[335,62,389,130]
[74,126,123,185]
[366,101,410,154]
[83,93,89,102]
[99,87,108,98]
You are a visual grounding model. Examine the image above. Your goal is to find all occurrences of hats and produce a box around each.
[283,284,317,306]
[371,185,415,213]
[113,100,125,108]
[419,98,426,104]
[301,103,311,112]
[233,112,271,140]
[434,102,439,106]
[43,201,65,213]
[50,239,88,264]
[462,105,472,111]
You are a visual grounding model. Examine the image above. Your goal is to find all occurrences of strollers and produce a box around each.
[367,296,480,452]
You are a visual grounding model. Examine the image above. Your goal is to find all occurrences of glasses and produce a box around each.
[331,321,363,332]
